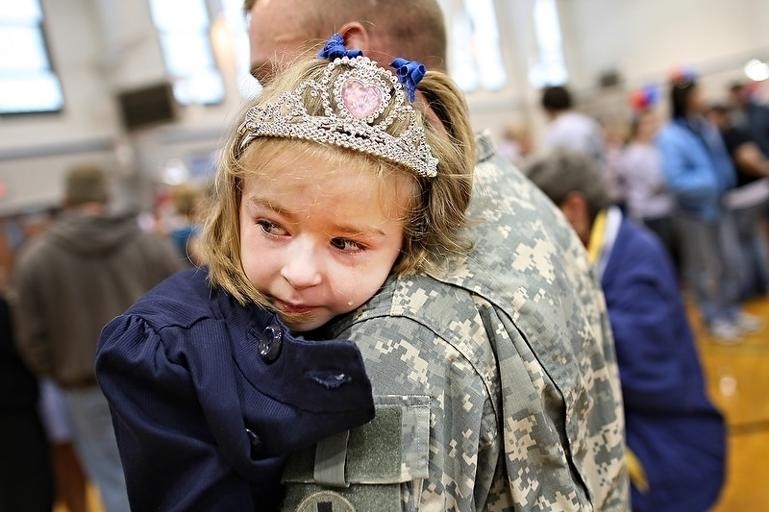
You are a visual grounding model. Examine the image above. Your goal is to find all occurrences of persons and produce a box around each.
[0,0,769,512]
